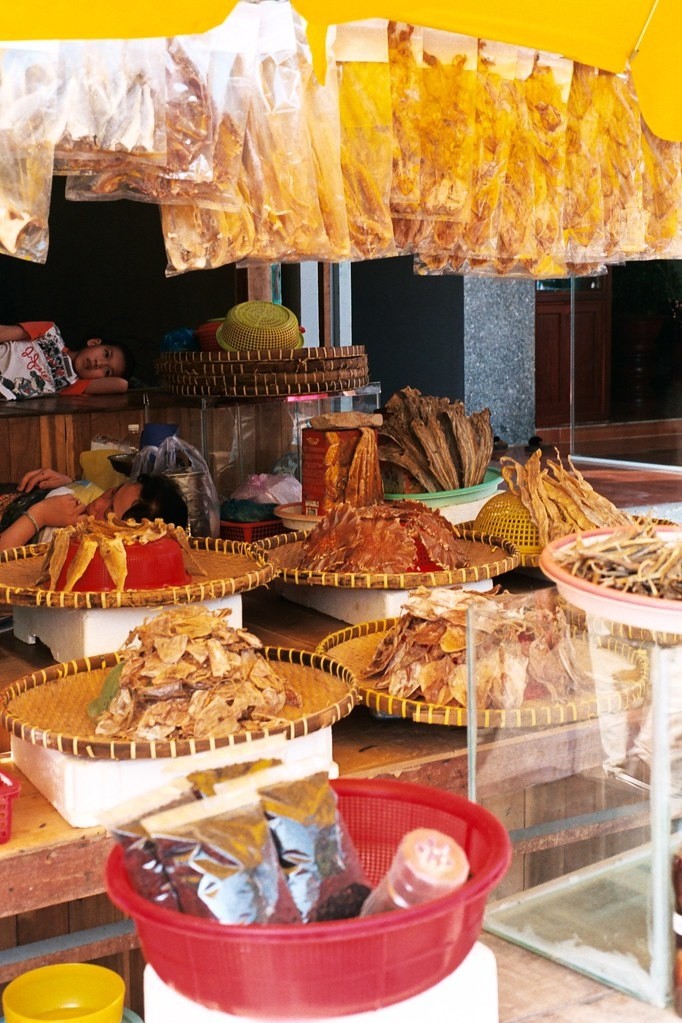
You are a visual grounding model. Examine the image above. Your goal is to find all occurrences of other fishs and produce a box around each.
[372,385,495,494]
[499,447,682,602]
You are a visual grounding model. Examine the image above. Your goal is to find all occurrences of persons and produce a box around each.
[0,322,128,403]
[0,467,189,552]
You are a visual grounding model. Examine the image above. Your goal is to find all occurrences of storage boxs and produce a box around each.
[10,728,340,833]
[466,604,681,1014]
[264,576,494,626]
[12,595,244,664]
[437,489,509,523]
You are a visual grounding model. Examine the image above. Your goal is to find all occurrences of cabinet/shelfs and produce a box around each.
[536,289,613,426]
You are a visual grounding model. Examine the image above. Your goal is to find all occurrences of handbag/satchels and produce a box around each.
[230,473,302,506]
[132,435,220,538]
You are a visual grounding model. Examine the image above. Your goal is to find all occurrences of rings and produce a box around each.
[40,471,44,475]
[75,499,81,505]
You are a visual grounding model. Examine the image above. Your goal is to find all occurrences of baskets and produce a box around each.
[160,299,369,397]
[552,594,682,646]
[455,520,543,568]
[318,621,649,728]
[472,491,552,553]
[254,529,521,588]
[0,649,357,760]
[103,781,513,1021]
[221,521,286,543]
[0,771,22,844]
[43,532,192,589]
[0,536,277,608]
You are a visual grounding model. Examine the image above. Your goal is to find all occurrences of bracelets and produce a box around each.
[23,511,39,533]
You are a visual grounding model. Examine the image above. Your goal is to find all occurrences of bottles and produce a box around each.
[357,829,470,917]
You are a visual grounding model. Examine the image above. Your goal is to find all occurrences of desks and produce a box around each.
[0,588,650,1011]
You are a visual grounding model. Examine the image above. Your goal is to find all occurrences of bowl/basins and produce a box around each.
[2,963,126,1023]
[539,525,682,633]
